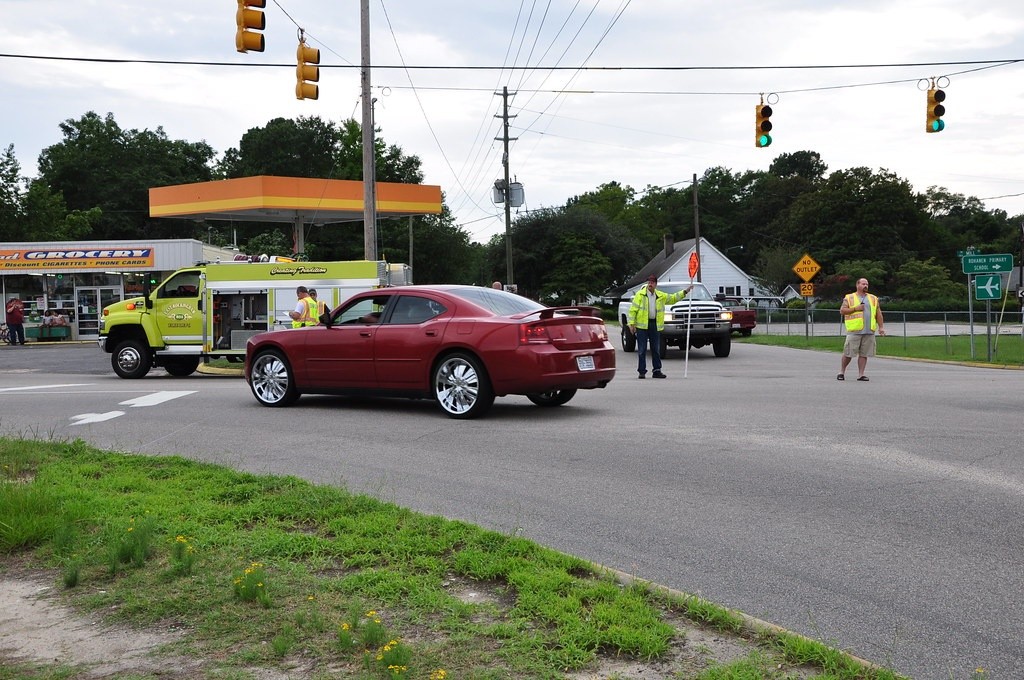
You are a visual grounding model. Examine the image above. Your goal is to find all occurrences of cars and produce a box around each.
[245,283,615,418]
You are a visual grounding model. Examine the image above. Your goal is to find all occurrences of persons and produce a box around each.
[40,310,53,337]
[365,297,409,323]
[492,281,502,290]
[289,286,320,329]
[309,289,330,324]
[51,310,67,326]
[6,298,29,345]
[627,275,694,379]
[837,278,886,381]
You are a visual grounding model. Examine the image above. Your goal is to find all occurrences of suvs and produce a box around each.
[619,281,733,357]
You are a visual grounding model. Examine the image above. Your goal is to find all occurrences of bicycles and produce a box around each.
[0,322,20,343]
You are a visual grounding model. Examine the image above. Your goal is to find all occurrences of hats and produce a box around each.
[646,274,657,282]
[309,288,316,295]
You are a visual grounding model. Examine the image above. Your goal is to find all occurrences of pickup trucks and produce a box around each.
[712,296,757,337]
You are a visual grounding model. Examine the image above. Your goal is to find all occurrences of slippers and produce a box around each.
[837,374,844,380]
[857,376,869,381]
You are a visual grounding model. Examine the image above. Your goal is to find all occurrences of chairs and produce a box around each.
[406,306,434,324]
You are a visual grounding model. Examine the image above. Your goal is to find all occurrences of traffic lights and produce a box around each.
[296,44,320,100]
[236,0,266,53]
[926,89,945,133]
[756,105,772,148]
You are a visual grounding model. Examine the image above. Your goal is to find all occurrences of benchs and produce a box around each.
[24,327,71,341]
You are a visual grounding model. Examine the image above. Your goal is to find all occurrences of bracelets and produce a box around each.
[684,290,687,294]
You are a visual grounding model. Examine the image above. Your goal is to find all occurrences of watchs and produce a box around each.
[854,308,855,311]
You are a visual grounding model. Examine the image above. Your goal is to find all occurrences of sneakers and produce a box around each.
[652,371,666,378]
[639,373,645,379]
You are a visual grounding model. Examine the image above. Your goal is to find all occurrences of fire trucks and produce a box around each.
[97,254,413,382]
